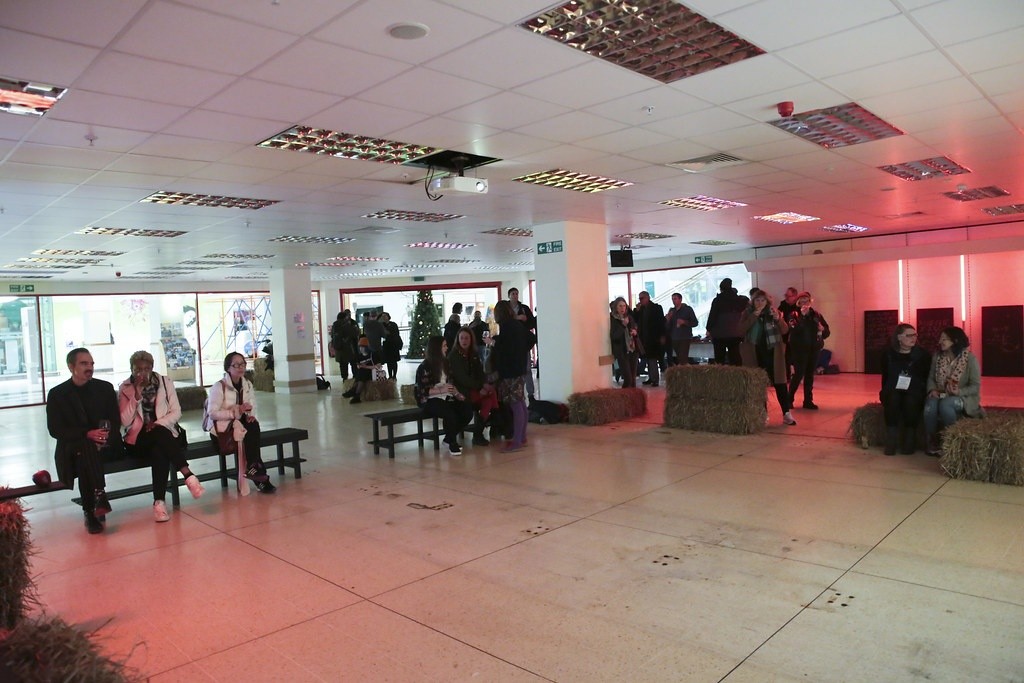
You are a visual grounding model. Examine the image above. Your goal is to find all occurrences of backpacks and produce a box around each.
[201,380,225,432]
[316,375,331,391]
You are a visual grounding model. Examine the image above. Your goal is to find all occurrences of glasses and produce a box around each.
[229,362,249,369]
[938,337,951,342]
[900,332,918,338]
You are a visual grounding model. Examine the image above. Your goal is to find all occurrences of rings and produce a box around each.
[103,437,105,439]
[99,437,102,439]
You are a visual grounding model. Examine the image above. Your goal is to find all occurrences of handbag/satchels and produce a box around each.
[175,421,188,457]
[217,431,241,457]
[398,336,403,350]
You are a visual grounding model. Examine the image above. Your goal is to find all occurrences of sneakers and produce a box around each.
[242,465,270,482]
[783,411,797,425]
[254,481,277,493]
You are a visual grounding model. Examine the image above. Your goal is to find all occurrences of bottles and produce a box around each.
[801,298,814,316]
[816,322,822,342]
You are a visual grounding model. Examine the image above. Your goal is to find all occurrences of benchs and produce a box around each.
[344,379,399,400]
[115,386,207,411]
[224,370,254,384]
[401,384,417,405]
[71,428,309,522]
[570,387,647,425]
[847,403,1024,486]
[364,401,504,459]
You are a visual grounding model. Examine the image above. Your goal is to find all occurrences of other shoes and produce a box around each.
[349,394,361,404]
[342,388,356,398]
[184,476,206,499]
[803,400,819,410]
[152,501,170,522]
[442,429,529,455]
[616,369,621,382]
[643,378,651,385]
[388,377,396,384]
[649,381,660,387]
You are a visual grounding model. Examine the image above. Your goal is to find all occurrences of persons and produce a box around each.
[482,300,530,452]
[667,293,699,366]
[633,291,664,386]
[610,298,646,387]
[46,348,122,534]
[507,287,536,402]
[469,311,490,346]
[118,351,204,521]
[444,302,462,346]
[737,287,797,425]
[206,352,277,493]
[415,327,491,455]
[707,278,747,365]
[331,307,403,403]
[923,327,985,453]
[786,291,831,410]
[777,286,798,321]
[879,322,933,455]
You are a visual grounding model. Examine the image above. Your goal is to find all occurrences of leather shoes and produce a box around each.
[83,511,103,535]
[90,488,112,517]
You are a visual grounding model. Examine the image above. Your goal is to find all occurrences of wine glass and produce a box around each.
[770,309,777,328]
[98,420,111,448]
[447,382,454,401]
[482,330,490,351]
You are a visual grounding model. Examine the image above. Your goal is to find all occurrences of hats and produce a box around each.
[358,337,369,346]
[794,291,811,300]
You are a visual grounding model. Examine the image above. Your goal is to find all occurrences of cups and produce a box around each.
[244,402,251,413]
[137,372,149,386]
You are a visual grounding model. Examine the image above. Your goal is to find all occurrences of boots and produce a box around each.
[900,422,914,455]
[926,434,938,455]
[884,423,898,455]
